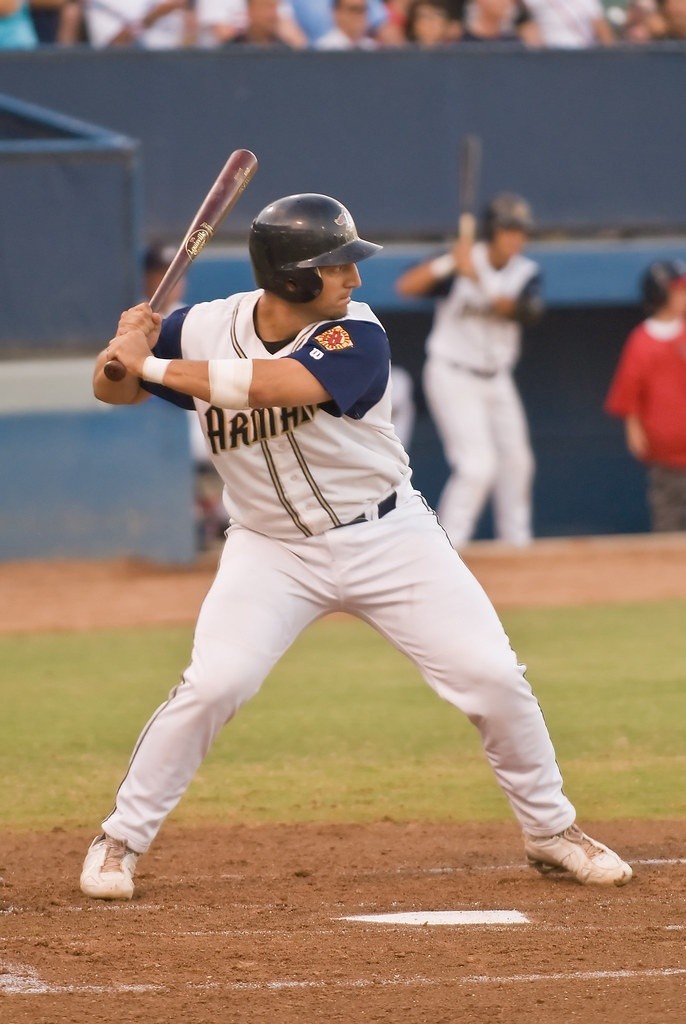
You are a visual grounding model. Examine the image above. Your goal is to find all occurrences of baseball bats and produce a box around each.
[450,132,478,268]
[102,148,262,381]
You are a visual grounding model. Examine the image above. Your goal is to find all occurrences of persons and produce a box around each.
[390,194,686,560]
[137,233,225,554]
[0,0,686,50]
[71,195,634,900]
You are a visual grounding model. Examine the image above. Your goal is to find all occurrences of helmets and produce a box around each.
[145,245,178,270]
[485,192,537,235]
[249,193,383,303]
[643,260,686,306]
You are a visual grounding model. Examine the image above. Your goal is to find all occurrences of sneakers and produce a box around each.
[80,836,140,900]
[525,823,633,887]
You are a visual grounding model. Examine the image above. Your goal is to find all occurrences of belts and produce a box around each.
[357,492,397,519]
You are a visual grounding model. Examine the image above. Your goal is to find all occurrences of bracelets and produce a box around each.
[142,356,173,385]
